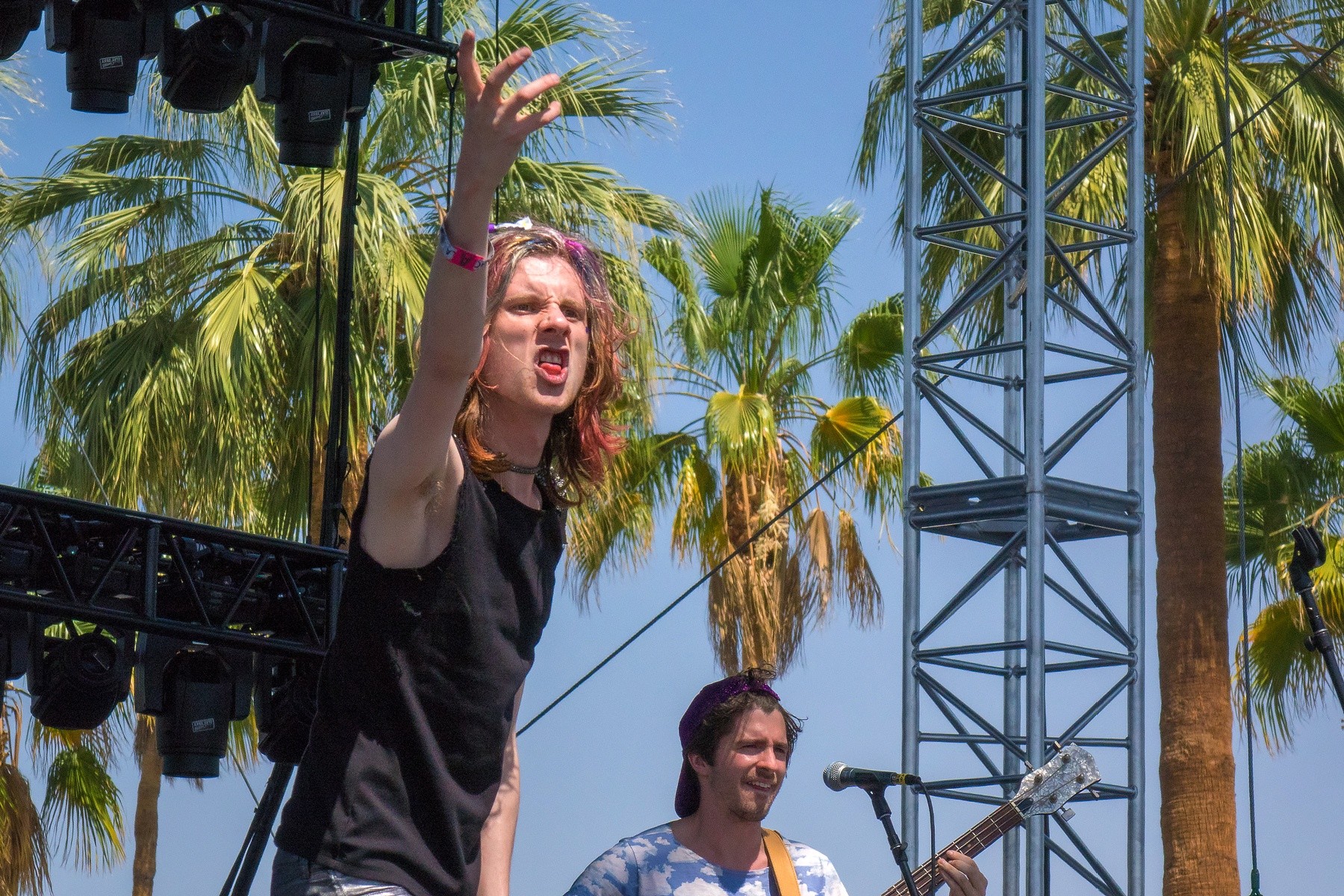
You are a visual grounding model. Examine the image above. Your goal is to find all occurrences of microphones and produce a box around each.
[822,759,922,793]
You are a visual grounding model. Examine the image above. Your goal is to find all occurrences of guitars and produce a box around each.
[879,739,1102,896]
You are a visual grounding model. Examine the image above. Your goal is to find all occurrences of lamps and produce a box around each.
[0,1,376,169]
[2,606,319,779]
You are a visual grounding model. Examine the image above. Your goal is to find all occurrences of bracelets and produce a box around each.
[440,214,496,271]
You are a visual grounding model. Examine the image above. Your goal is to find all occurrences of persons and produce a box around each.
[272,31,638,896]
[561,666,988,896]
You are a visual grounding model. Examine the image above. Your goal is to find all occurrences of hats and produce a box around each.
[674,674,781,818]
